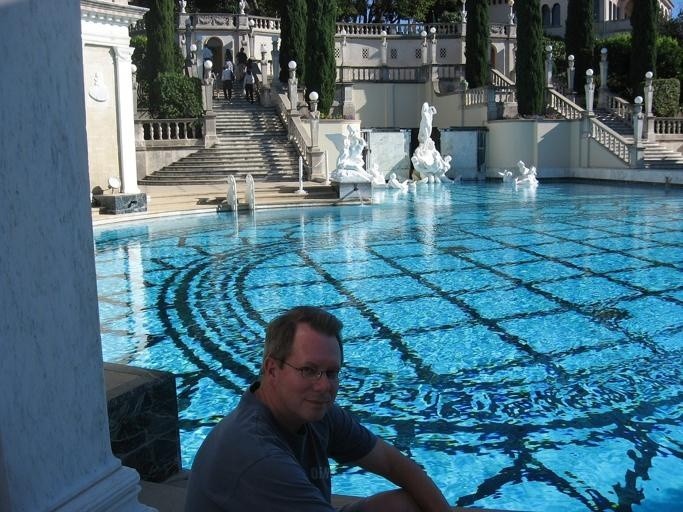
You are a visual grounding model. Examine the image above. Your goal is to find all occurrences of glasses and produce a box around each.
[277,356,347,384]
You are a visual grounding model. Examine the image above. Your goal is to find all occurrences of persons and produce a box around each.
[221,46,262,105]
[203,43,214,60]
[182,306,453,511]
